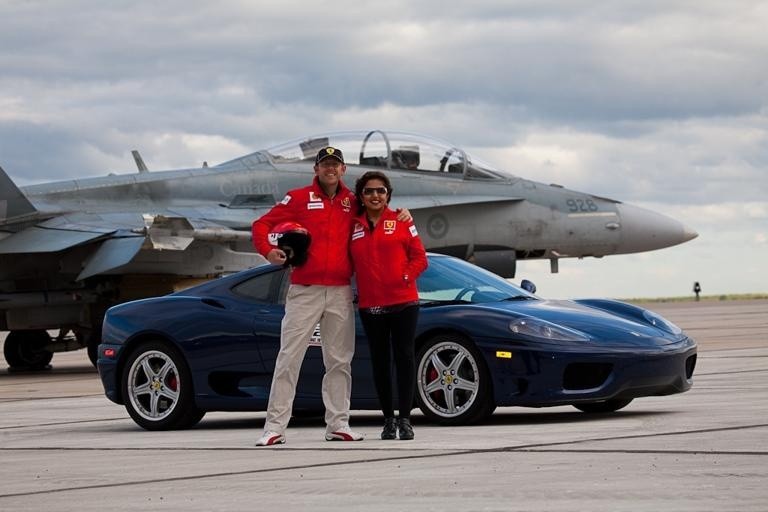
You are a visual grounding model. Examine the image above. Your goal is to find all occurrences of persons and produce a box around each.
[252,147,413,447]
[349,172,428,439]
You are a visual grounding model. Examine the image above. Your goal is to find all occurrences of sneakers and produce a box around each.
[325,424,364,442]
[256,429,287,446]
[380,417,415,440]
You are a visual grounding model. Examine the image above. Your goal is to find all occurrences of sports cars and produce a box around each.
[96,247,697,434]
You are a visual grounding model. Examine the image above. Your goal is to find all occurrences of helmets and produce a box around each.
[267,220,311,267]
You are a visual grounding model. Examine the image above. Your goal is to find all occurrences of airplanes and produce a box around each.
[0,123,703,385]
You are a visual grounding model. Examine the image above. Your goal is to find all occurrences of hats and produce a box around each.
[315,147,344,166]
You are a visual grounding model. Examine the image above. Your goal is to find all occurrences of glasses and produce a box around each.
[362,186,388,196]
